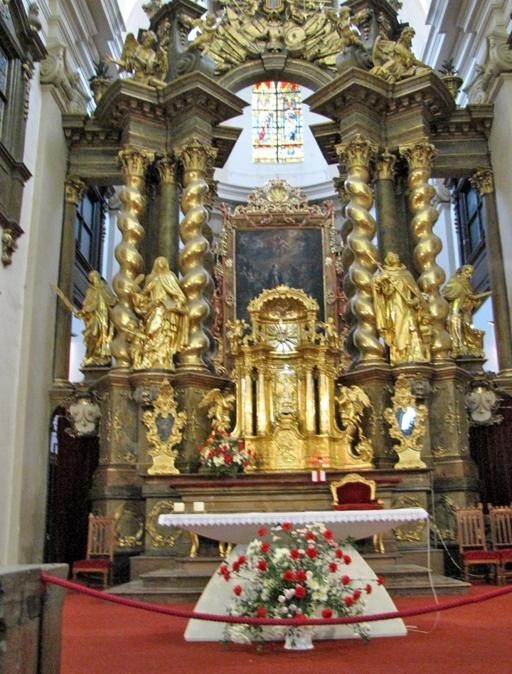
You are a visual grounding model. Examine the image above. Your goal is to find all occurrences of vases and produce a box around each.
[281,616,318,649]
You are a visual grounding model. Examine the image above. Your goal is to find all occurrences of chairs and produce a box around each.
[455,502,512,587]
[328,472,387,553]
[72,511,119,593]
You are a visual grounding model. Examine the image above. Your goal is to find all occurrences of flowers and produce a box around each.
[214,521,383,653]
[194,424,258,480]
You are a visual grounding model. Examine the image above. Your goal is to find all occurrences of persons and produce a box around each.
[73,268,117,369]
[372,251,431,367]
[334,6,365,56]
[184,8,218,61]
[368,22,430,81]
[102,29,167,88]
[126,255,189,370]
[443,263,492,351]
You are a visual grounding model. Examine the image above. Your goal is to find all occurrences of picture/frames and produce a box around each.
[212,178,343,372]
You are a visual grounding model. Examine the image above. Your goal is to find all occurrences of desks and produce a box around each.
[157,507,439,642]
